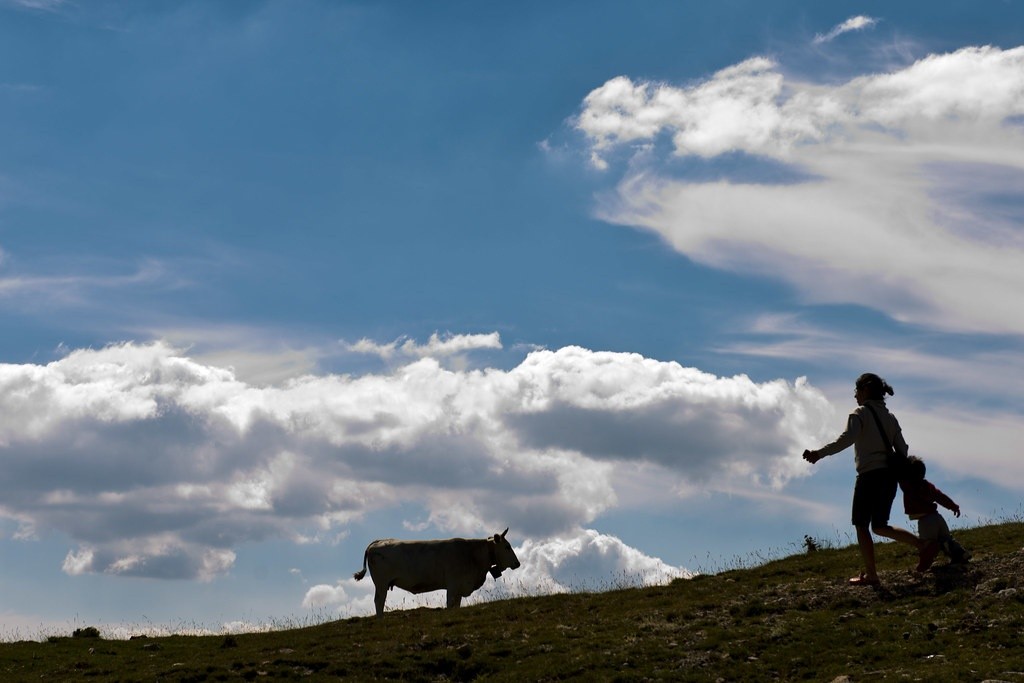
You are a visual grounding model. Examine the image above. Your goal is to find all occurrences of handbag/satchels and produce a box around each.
[885,453,910,482]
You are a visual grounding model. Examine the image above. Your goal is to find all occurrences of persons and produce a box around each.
[802,374,939,586]
[901,460,974,567]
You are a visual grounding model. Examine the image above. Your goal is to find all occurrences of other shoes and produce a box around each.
[916,538,939,572]
[849,571,880,585]
[950,551,972,564]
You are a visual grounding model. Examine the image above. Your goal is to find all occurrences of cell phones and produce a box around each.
[803,449,811,458]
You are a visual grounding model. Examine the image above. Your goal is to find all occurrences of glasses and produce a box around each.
[854,387,858,393]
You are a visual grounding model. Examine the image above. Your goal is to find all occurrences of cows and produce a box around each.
[353,526,520,615]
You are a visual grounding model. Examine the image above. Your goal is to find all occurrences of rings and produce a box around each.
[810,457,812,459]
